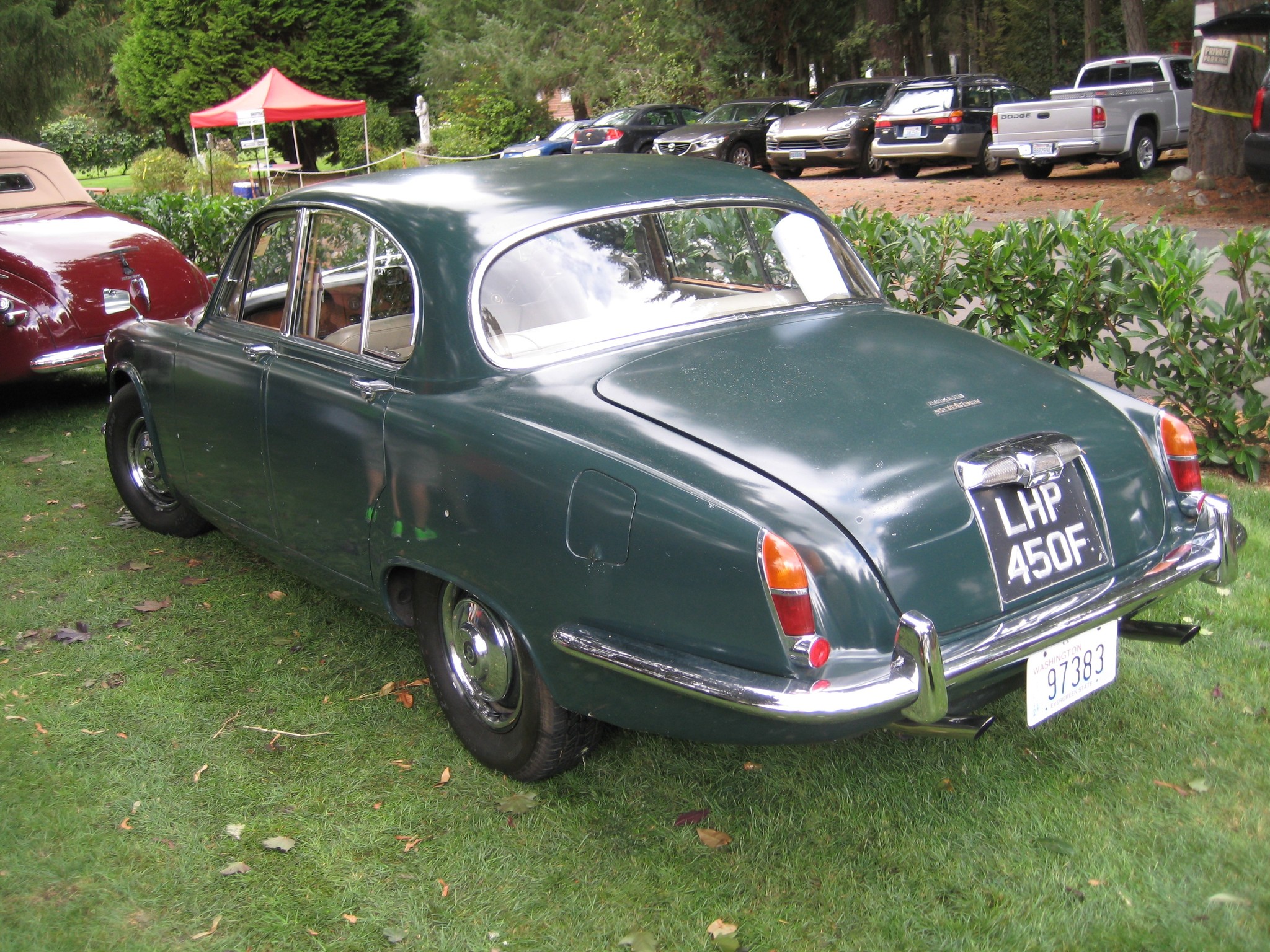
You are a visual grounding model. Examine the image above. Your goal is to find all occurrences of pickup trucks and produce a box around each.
[985,53,1196,181]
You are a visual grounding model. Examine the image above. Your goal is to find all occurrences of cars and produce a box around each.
[496,103,710,154]
[0,139,227,394]
[103,152,1249,781]
[651,96,819,172]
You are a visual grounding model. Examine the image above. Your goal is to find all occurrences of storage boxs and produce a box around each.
[232,180,260,201]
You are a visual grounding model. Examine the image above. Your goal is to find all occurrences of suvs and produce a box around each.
[766,74,922,179]
[1192,5,1270,183]
[871,74,1046,180]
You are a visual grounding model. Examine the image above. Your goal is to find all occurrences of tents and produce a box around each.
[190,67,370,196]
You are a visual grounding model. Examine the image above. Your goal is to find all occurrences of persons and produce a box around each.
[415,96,430,143]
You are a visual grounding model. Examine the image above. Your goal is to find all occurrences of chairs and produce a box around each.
[656,111,675,125]
[267,161,293,196]
[321,262,807,360]
[848,92,865,106]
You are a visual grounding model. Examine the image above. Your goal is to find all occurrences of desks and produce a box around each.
[247,163,302,196]
